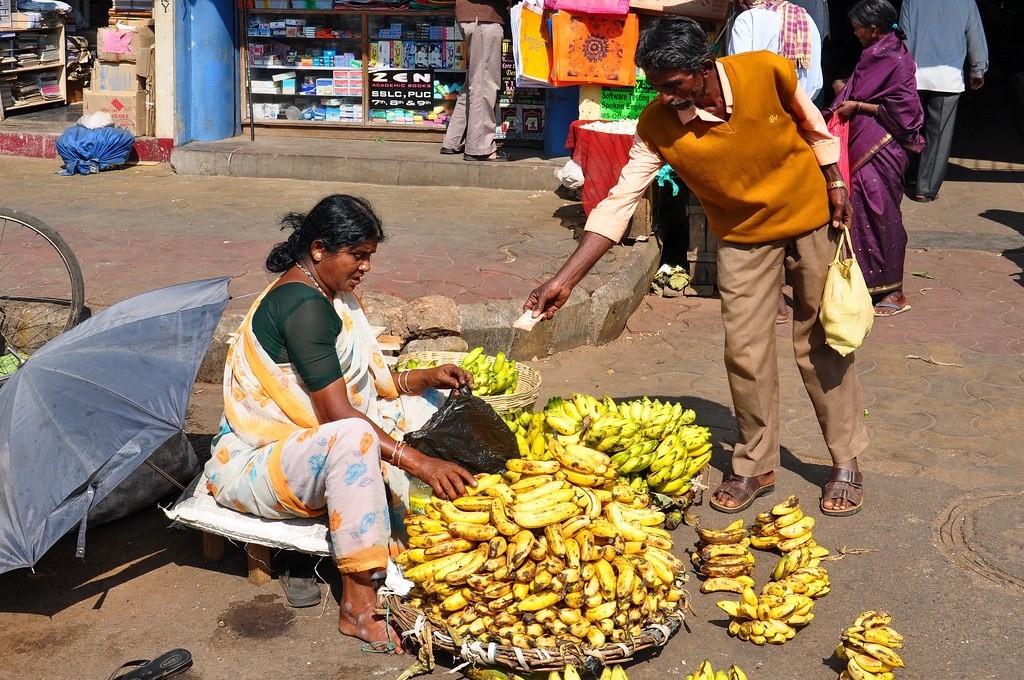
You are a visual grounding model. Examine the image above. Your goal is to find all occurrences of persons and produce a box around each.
[522,14,874,516]
[441,0,518,161]
[202,194,474,655]
[900,0,989,203]
[821,0,928,317]
[727,0,823,102]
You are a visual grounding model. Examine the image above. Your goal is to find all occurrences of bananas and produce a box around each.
[836,610,906,680]
[493,660,750,680]
[396,346,519,396]
[692,495,832,645]
[394,393,711,650]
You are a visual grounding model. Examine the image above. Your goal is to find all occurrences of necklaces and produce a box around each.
[294,258,332,305]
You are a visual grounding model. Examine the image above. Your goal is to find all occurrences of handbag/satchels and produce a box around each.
[404,383,522,476]
[819,226,875,357]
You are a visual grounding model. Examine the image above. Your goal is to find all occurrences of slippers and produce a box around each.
[107,648,195,680]
[711,473,775,513]
[874,302,911,316]
[821,468,863,516]
[278,556,323,608]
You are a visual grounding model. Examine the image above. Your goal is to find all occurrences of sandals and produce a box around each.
[917,194,940,202]
[464,152,515,161]
[441,147,464,154]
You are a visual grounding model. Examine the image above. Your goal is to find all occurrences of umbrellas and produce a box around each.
[1,275,233,576]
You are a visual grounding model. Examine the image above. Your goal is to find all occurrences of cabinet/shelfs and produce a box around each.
[239,9,467,133]
[495,37,546,147]
[0,23,68,121]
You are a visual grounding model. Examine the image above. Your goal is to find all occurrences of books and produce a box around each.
[0,32,63,108]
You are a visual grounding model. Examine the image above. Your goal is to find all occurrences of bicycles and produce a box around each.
[1,208,81,376]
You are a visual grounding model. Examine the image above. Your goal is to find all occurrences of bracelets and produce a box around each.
[827,180,848,191]
[398,369,413,394]
[390,440,408,468]
[856,101,863,112]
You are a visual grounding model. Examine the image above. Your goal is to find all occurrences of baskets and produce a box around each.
[397,350,543,413]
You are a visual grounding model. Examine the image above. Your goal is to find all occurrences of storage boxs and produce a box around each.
[583,159,718,294]
[246,0,467,125]
[0,0,155,137]
